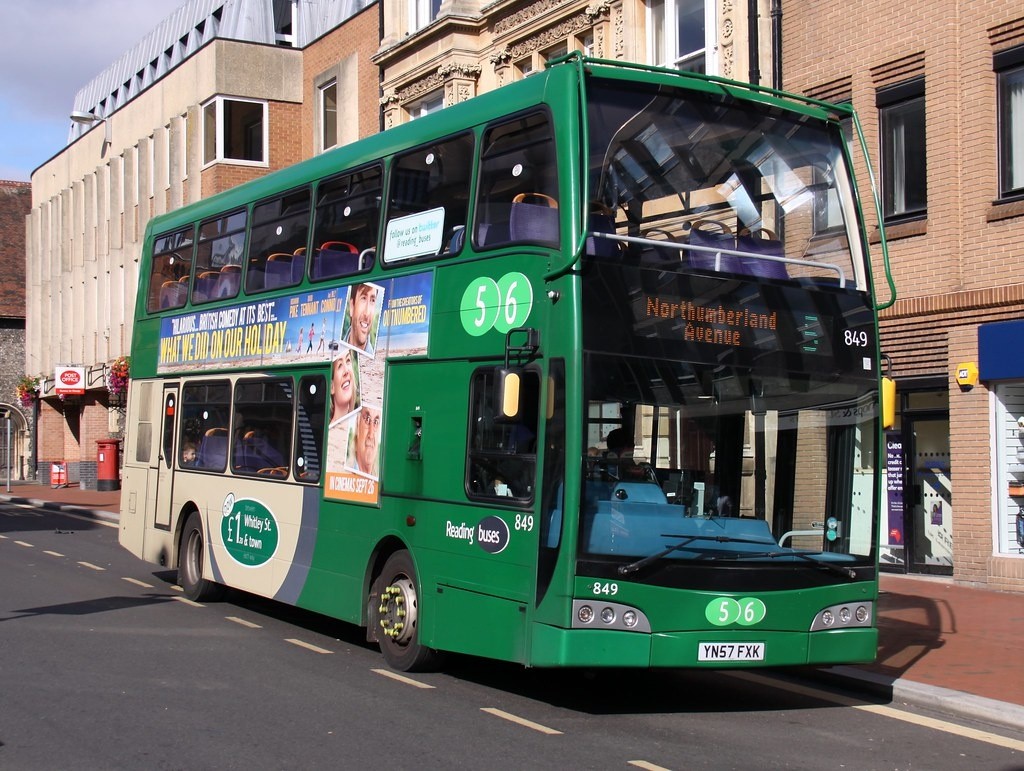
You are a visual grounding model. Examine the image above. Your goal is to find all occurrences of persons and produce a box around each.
[319,318,326,353]
[307,323,315,352]
[356,406,381,475]
[235,411,242,434]
[343,283,378,354]
[606,428,657,488]
[331,350,356,424]
[297,327,304,351]
[494,401,565,499]
[181,438,198,463]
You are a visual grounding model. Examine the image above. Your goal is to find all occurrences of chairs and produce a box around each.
[193,428,308,479]
[157,192,791,311]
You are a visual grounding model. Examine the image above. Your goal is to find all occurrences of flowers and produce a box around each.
[106,356,131,394]
[15,375,42,409]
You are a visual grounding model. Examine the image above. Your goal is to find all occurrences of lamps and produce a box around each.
[70,112,112,143]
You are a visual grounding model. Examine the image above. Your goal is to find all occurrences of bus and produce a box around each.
[110,51,901,674]
